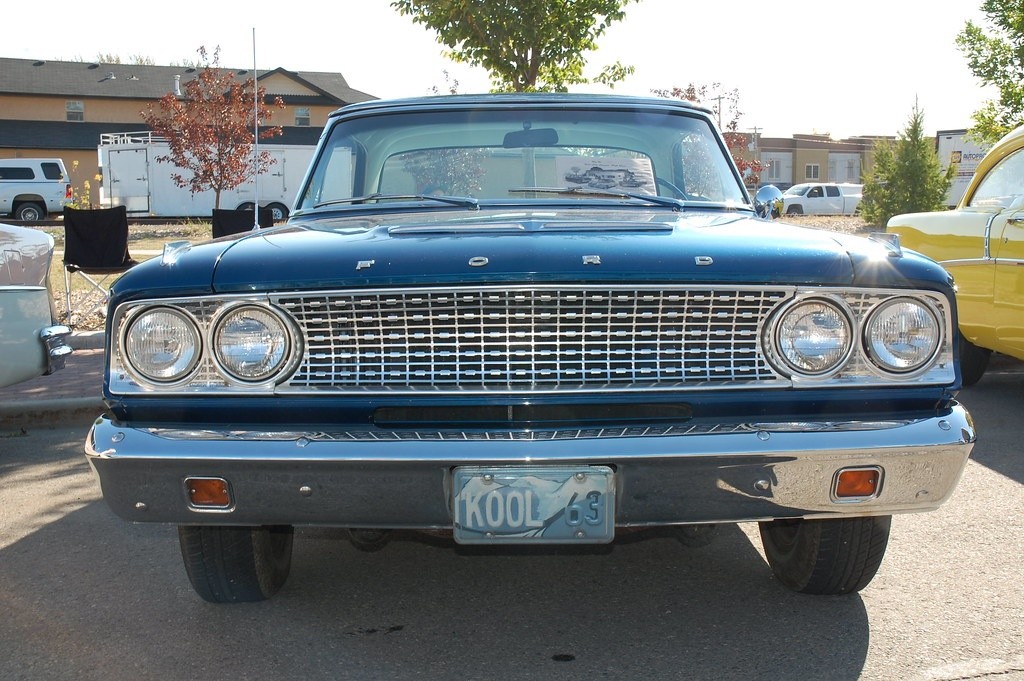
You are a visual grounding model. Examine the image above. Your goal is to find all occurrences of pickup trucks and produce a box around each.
[782,183,864,218]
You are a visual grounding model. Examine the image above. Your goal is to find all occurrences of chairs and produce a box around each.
[61,206,139,331]
[212,209,274,240]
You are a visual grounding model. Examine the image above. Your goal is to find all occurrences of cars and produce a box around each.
[84,94,978,605]
[886,124,1023,391]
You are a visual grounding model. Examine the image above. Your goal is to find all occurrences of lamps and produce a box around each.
[105,72,117,80]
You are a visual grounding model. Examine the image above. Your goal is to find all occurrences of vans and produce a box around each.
[0,157,74,223]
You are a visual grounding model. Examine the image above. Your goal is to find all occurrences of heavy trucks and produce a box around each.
[98,131,417,229]
